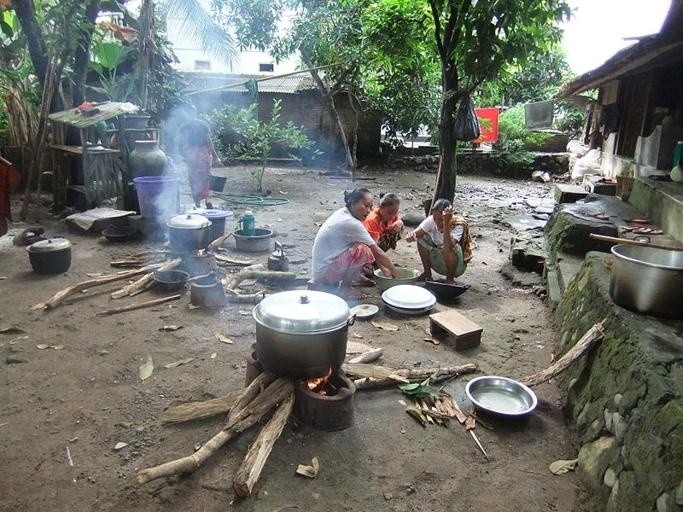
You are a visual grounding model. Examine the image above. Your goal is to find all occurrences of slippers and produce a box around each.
[205,202,213,210]
[352,276,377,286]
[338,288,368,300]
[417,273,432,282]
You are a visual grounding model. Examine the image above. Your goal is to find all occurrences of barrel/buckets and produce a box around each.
[131,175,180,219]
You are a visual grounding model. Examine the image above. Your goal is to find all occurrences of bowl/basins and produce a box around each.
[464,374,538,416]
[166,212,213,252]
[232,227,273,253]
[374,264,421,290]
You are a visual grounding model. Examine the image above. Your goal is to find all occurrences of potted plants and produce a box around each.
[616,159,645,197]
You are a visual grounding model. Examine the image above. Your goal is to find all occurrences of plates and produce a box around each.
[380,286,438,310]
[351,304,380,319]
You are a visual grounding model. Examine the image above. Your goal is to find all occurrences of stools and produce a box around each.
[427,308,485,350]
[307,277,339,295]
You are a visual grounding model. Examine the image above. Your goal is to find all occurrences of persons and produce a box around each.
[312,188,401,300]
[363,191,405,277]
[174,103,220,209]
[404,199,473,283]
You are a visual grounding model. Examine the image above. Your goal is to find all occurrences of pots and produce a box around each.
[101,222,140,243]
[425,278,471,302]
[152,268,189,292]
[610,234,683,316]
[251,290,357,381]
[25,236,72,274]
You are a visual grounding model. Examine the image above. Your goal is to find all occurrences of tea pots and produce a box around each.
[267,239,289,273]
